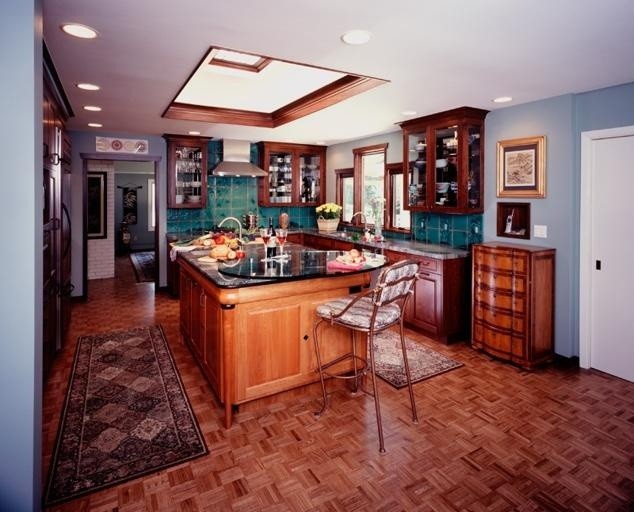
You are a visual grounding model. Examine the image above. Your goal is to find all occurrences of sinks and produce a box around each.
[191,247,213,256]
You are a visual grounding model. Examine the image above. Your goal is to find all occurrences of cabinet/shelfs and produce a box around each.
[61,126,74,335]
[302,232,354,252]
[393,105,492,215]
[42,89,62,369]
[274,232,304,247]
[468,239,557,374]
[254,140,328,209]
[382,246,470,347]
[162,132,214,210]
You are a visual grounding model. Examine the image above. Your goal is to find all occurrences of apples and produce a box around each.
[350,248,360,258]
[216,236,226,245]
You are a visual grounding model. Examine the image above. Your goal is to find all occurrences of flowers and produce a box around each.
[315,201,343,219]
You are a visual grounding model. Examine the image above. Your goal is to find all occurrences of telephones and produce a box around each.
[505,209,514,232]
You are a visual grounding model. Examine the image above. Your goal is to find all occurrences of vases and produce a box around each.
[315,217,340,232]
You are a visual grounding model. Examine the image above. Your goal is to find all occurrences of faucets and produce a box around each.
[353,212,367,229]
[218,216,243,242]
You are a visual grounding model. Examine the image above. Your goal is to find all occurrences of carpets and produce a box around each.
[128,248,159,285]
[41,323,211,511]
[361,329,467,390]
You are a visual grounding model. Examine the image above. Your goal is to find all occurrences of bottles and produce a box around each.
[267,218,276,261]
[408,184,416,206]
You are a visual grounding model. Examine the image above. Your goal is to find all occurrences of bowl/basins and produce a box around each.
[191,182,201,187]
[436,183,450,189]
[185,182,191,187]
[307,165,318,169]
[188,196,200,205]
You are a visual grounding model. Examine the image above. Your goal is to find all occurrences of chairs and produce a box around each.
[311,258,424,455]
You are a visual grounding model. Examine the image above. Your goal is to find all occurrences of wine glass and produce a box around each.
[259,228,272,262]
[275,229,288,259]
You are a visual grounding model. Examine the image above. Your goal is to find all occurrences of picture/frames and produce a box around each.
[496,201,532,241]
[495,134,546,199]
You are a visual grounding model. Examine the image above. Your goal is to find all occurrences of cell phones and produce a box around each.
[504,209,515,233]
